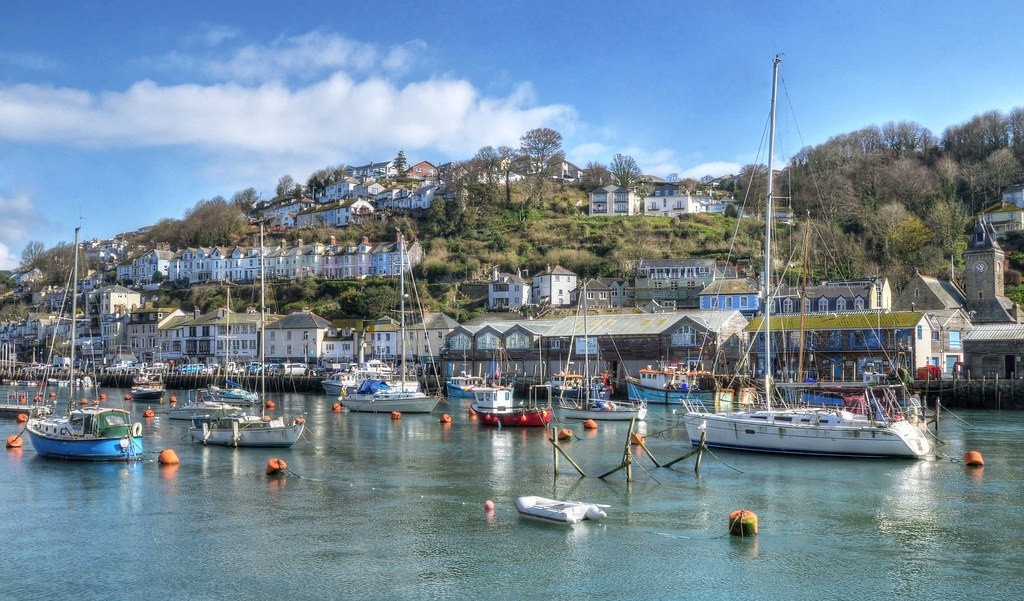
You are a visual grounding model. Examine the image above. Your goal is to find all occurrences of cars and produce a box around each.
[17,356,441,379]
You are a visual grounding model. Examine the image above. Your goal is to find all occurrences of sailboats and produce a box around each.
[189,220,305,447]
[196,286,260,407]
[340,234,442,413]
[557,277,647,421]
[25,225,144,463]
[682,51,932,459]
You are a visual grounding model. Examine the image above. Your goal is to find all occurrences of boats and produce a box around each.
[626,346,755,405]
[469,385,553,428]
[130,371,166,401]
[445,373,515,402]
[513,495,611,525]
[166,399,247,420]
[544,373,612,401]
[320,361,420,397]
